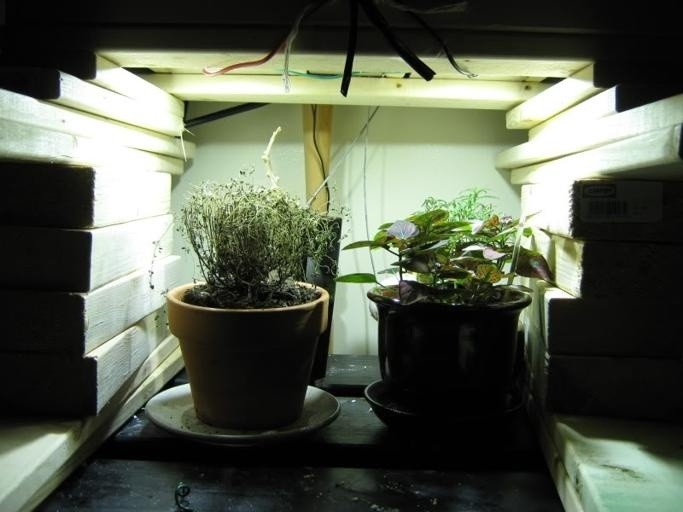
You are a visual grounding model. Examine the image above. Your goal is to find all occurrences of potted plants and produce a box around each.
[344,185,542,429]
[160,173,337,427]
